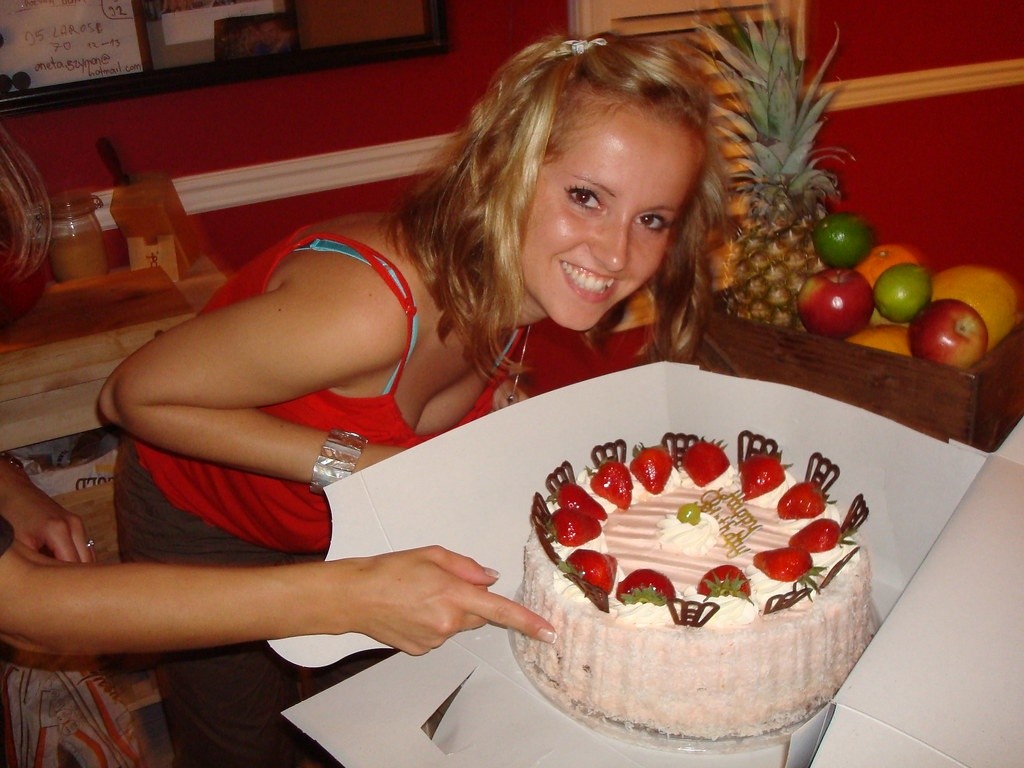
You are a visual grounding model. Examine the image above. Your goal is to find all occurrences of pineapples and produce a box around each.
[722,217,816,329]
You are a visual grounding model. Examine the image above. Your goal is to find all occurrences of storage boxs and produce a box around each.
[783,417,1024,768]
[696,272,1024,454]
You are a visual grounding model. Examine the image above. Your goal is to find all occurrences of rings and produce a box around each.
[86,540,94,548]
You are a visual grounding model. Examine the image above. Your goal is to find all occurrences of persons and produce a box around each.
[0,451,558,768]
[99,32,724,767]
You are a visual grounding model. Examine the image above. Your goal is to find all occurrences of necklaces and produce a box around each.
[498,324,530,405]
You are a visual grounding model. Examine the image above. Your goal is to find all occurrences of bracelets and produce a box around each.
[310,427,368,493]
[0,452,23,468]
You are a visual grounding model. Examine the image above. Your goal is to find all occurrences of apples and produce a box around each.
[912,300,987,368]
[796,271,876,338]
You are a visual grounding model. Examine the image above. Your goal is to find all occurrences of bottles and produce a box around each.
[46,189,108,284]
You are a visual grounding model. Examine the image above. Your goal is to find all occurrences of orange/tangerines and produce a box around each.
[842,325,910,356]
[936,266,1016,349]
[857,243,914,286]
[815,213,872,268]
[872,262,931,322]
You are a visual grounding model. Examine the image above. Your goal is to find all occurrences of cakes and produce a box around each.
[514,443,880,738]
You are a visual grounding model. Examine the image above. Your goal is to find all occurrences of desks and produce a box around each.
[0,282,203,768]
